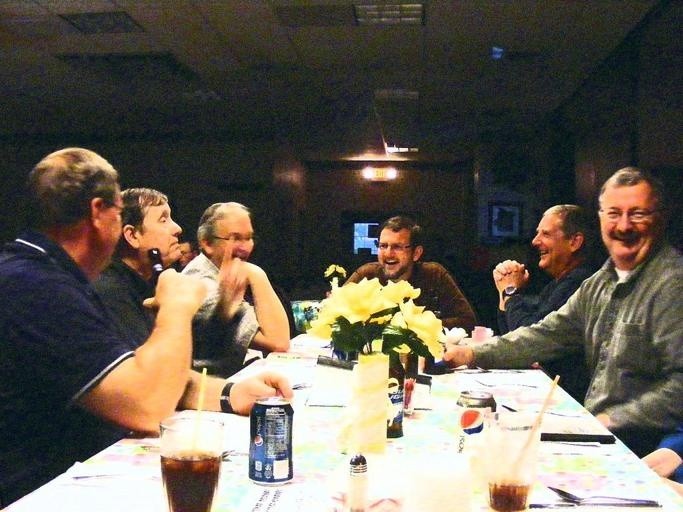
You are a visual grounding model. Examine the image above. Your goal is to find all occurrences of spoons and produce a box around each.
[548,487,658,505]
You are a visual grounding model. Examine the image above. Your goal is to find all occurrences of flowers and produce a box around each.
[324,264,347,279]
[307,277,445,374]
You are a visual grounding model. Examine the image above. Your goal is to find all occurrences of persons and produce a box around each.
[90,188,250,362]
[179,199,291,360]
[1,147,209,511]
[638,425,683,497]
[493,203,599,396]
[178,239,199,267]
[341,215,476,336]
[443,169,683,433]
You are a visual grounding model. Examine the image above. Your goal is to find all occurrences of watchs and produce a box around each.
[502,285,518,300]
[219,382,233,413]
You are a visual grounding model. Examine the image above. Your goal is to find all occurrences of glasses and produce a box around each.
[376,242,411,252]
[595,206,661,222]
[212,234,259,244]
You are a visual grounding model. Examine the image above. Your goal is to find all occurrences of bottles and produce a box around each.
[345,452,372,512]
[452,391,497,467]
[384,351,406,438]
[248,397,295,487]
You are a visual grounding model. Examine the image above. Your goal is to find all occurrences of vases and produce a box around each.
[356,351,389,455]
[332,276,338,292]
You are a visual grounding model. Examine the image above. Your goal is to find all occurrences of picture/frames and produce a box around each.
[488,199,524,241]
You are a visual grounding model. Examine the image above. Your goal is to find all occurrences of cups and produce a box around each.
[159,417,224,512]
[483,413,540,512]
